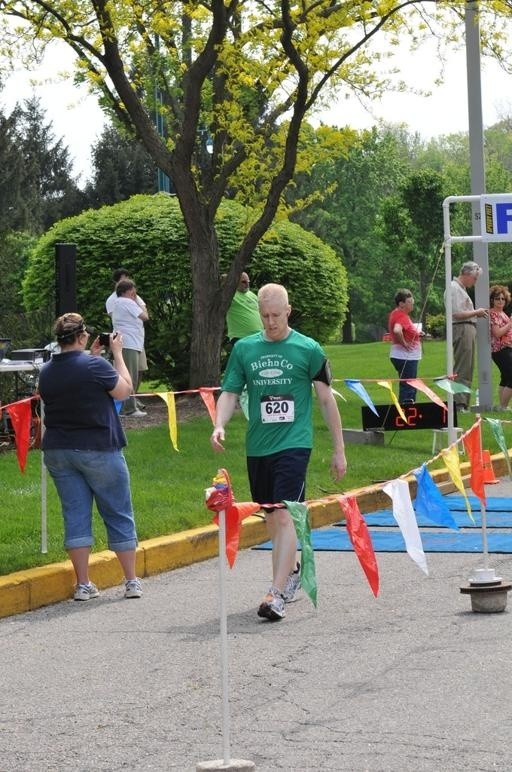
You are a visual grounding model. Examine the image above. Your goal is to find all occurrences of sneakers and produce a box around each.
[256,586,287,618]
[283,561,303,603]
[125,408,147,417]
[73,580,100,601]
[124,577,143,598]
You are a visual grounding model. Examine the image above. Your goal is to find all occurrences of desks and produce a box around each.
[1,363,41,449]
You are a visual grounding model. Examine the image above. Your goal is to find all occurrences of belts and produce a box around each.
[453,321,476,326]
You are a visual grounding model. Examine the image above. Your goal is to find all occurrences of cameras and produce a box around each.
[100,333,117,346]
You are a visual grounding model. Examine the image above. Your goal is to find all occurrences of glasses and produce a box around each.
[494,297,505,300]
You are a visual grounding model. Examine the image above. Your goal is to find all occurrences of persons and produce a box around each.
[210,283,347,619]
[219,272,264,345]
[444,261,489,413]
[39,312,143,601]
[490,285,512,412]
[389,288,425,404]
[105,268,150,417]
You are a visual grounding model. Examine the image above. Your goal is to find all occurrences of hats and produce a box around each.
[55,320,97,339]
